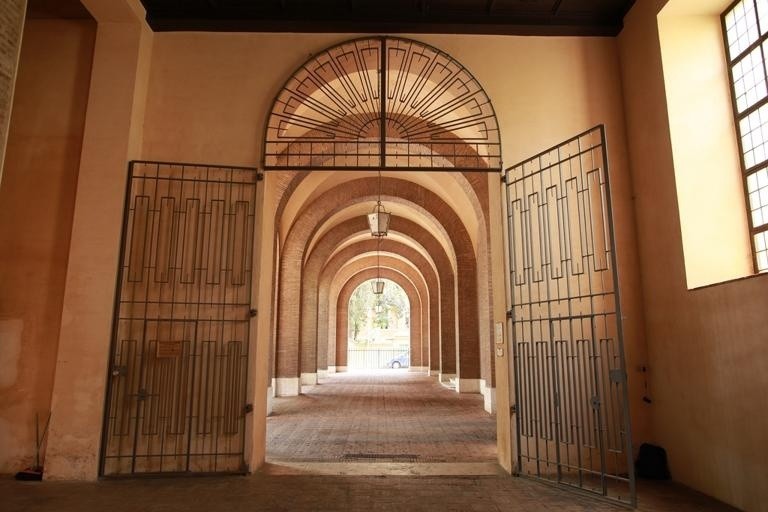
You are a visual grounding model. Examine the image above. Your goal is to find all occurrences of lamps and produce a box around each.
[365,172,393,293]
